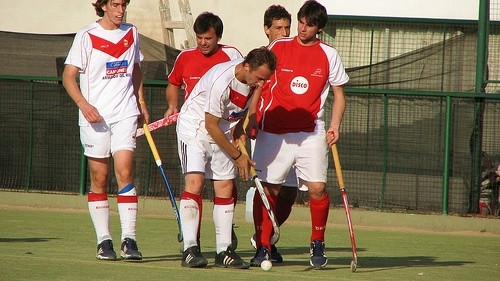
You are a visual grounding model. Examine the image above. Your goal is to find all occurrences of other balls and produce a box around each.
[260,260,272,271]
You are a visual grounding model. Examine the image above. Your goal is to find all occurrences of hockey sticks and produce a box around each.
[140,115,184,243]
[327,130,358,273]
[236,138,281,245]
[135,112,180,138]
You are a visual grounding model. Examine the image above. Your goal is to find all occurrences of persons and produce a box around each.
[163,12,244,253]
[62,0,150,260]
[250,4,297,262]
[246,0,350,267]
[176,46,278,268]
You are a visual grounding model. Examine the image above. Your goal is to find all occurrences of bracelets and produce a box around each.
[232,151,241,161]
[137,101,145,105]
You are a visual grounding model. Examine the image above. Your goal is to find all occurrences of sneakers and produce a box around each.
[250,247,270,267]
[310,239,327,269]
[215,246,250,269]
[181,245,209,267]
[270,244,284,264]
[121,237,142,259]
[231,224,237,249]
[97,240,116,260]
[250,233,258,249]
